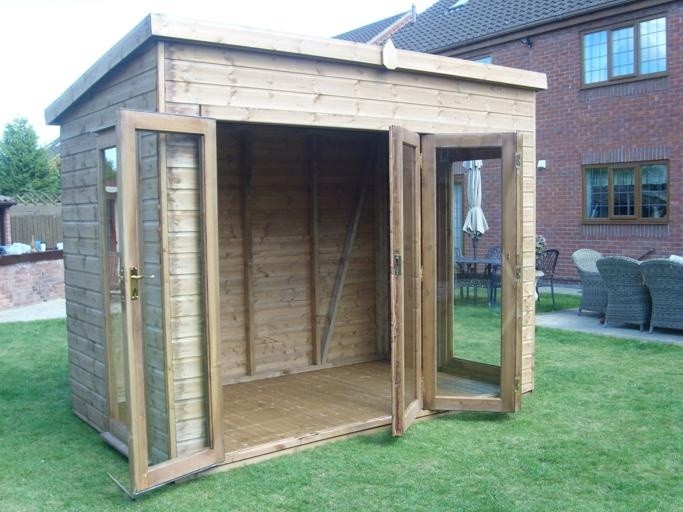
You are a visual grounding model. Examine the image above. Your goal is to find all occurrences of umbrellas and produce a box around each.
[460,158,490,298]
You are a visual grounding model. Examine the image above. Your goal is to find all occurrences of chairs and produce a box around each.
[636,256,682,333]
[451,234,562,308]
[572,248,609,323]
[595,254,652,331]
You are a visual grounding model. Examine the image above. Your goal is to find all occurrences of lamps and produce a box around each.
[519,36,532,48]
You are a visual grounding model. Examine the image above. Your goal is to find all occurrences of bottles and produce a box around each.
[31,235,34,252]
[589,202,599,218]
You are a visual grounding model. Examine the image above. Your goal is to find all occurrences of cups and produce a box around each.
[40,243,46,251]
[34,240,41,251]
[56,242,62,250]
